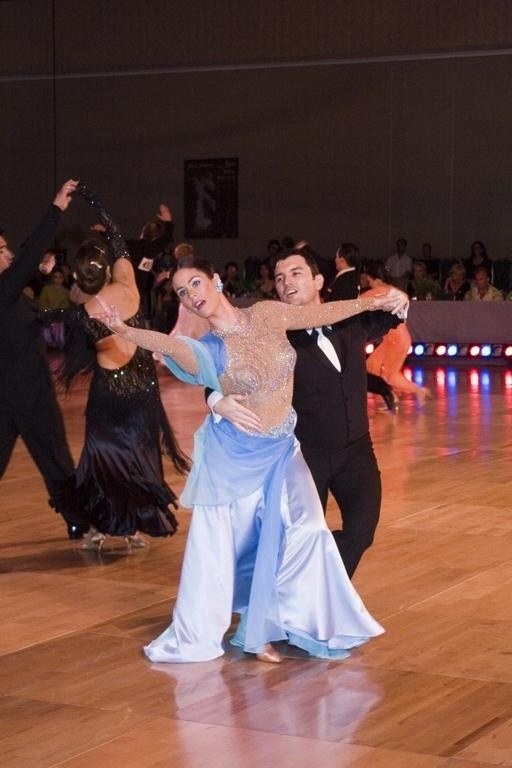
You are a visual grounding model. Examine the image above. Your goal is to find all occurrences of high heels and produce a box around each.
[126,536,149,553]
[78,530,106,553]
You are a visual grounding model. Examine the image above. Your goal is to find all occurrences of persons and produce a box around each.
[204,244,411,582]
[90,253,411,665]
[0,179,91,539]
[23,203,512,411]
[48,179,193,547]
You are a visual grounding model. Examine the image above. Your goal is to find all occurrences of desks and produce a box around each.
[227,297,511,368]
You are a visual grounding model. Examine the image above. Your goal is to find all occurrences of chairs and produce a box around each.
[242,255,511,298]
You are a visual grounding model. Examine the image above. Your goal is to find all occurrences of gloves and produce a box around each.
[77,182,130,263]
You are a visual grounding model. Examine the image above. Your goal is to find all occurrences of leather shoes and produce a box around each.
[69,522,90,537]
[255,643,282,663]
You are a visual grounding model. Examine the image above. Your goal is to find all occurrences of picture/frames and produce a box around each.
[182,156,240,242]
